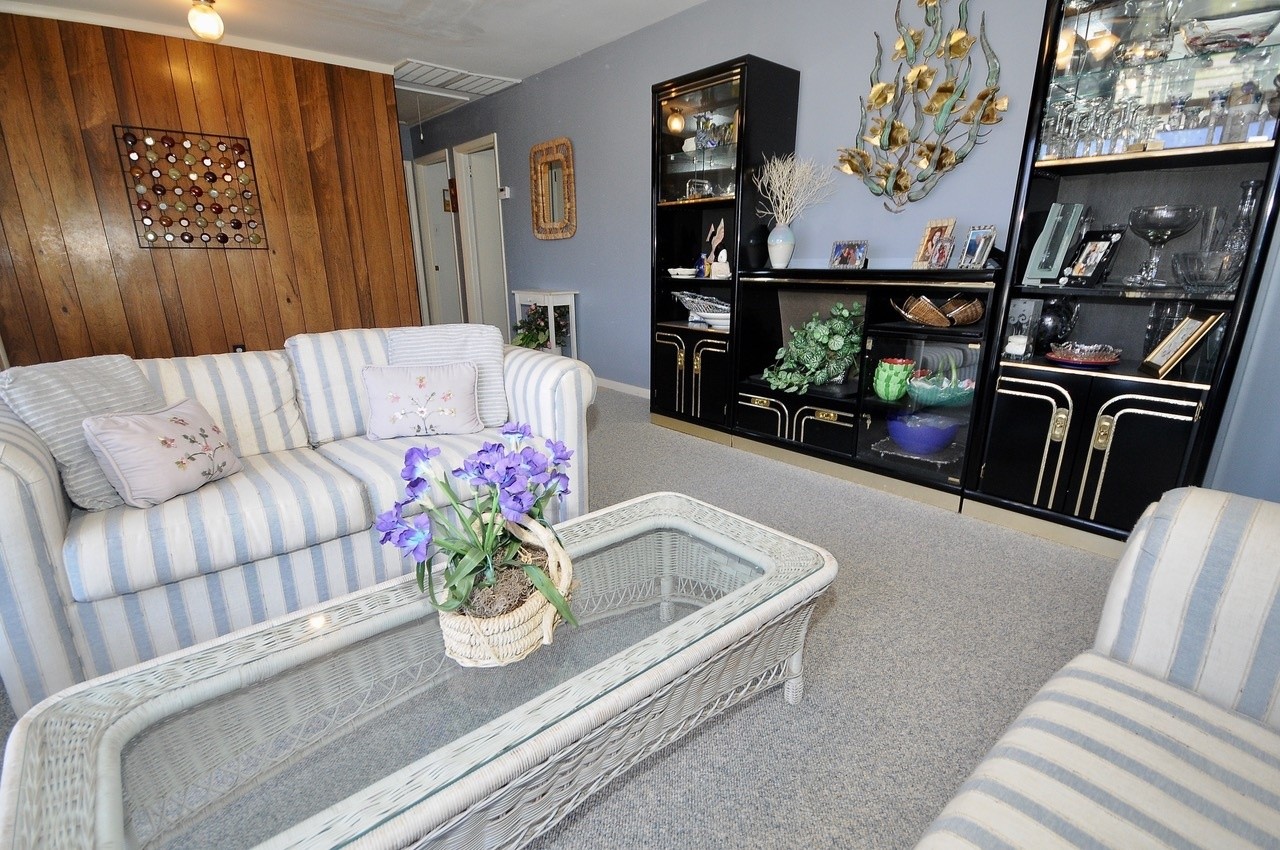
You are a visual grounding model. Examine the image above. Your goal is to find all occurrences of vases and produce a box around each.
[1209,180,1263,293]
[746,225,772,270]
[767,223,795,269]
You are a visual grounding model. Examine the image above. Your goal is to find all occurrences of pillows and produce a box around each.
[387,322,509,429]
[362,361,485,440]
[81,397,243,510]
[0,354,166,510]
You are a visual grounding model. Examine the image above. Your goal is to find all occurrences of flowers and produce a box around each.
[378,421,579,629]
[511,306,570,353]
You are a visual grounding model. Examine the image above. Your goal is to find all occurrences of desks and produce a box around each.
[514,289,579,361]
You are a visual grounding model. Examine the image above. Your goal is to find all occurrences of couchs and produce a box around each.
[908,488,1280,850]
[0,330,596,717]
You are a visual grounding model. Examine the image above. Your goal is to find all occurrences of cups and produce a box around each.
[1144,302,1194,357]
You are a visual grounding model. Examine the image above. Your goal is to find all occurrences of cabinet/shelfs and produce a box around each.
[651,0,1280,540]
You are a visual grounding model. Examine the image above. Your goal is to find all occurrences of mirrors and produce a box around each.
[530,138,576,240]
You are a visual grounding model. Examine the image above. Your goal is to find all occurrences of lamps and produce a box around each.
[188,0,225,40]
[666,106,685,135]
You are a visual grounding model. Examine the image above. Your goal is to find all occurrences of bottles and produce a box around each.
[1111,0,1184,67]
[1220,179,1263,280]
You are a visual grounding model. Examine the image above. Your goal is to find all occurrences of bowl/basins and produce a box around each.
[1175,253,1243,286]
[873,357,916,401]
[695,312,730,330]
[886,416,962,452]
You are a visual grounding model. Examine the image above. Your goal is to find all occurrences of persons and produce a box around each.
[937,245,947,264]
[1082,243,1100,275]
[837,248,856,265]
[924,227,942,261]
[965,233,981,264]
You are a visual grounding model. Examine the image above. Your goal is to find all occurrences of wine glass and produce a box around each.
[1047,95,1158,157]
[1121,204,1203,288]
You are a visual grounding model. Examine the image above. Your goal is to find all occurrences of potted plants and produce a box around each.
[762,305,864,393]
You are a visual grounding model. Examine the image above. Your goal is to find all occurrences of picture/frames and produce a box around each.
[927,236,955,269]
[912,218,956,270]
[828,240,868,269]
[1060,230,1125,289]
[958,225,994,269]
[1139,308,1226,381]
[970,232,996,269]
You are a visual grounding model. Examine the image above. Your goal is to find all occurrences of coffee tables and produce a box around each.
[0,492,839,850]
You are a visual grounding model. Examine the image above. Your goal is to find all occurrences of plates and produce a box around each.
[668,268,698,275]
[1045,343,1122,369]
